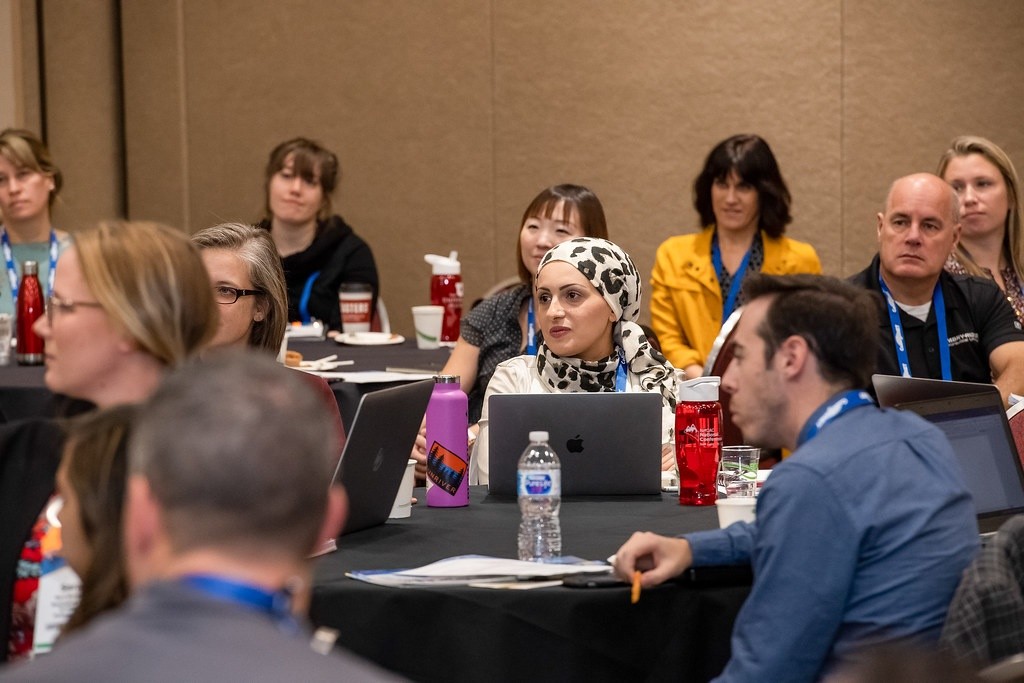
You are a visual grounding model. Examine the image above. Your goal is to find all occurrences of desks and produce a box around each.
[0,341,752,683]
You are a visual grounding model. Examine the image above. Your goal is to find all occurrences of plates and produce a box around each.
[335,331,406,346]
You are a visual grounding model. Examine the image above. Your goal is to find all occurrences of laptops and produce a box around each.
[872,373,1024,540]
[329,379,438,537]
[489,392,662,496]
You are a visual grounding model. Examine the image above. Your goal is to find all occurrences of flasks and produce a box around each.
[426,375,469,508]
[18,261,44,366]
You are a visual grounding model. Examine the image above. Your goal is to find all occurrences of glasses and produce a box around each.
[45,290,105,329]
[214,287,263,305]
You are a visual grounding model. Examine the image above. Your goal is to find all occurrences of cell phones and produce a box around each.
[563,573,631,588]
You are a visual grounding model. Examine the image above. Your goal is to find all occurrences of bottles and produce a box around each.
[424,250,465,346]
[518,431,562,569]
[674,375,722,505]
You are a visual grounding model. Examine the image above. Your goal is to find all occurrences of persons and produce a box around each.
[613,274,980,683]
[190,219,290,359]
[470,237,685,486]
[409,183,607,478]
[649,136,822,380]
[1,354,388,682]
[253,134,380,339]
[844,172,1024,413]
[42,220,222,408]
[1,129,72,371]
[936,137,1023,321]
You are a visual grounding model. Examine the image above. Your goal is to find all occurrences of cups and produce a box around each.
[411,305,445,350]
[721,445,761,500]
[338,283,373,342]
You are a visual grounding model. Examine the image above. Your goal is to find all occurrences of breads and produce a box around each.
[285,351,303,367]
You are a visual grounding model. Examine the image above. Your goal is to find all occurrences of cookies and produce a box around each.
[630,570,642,603]
